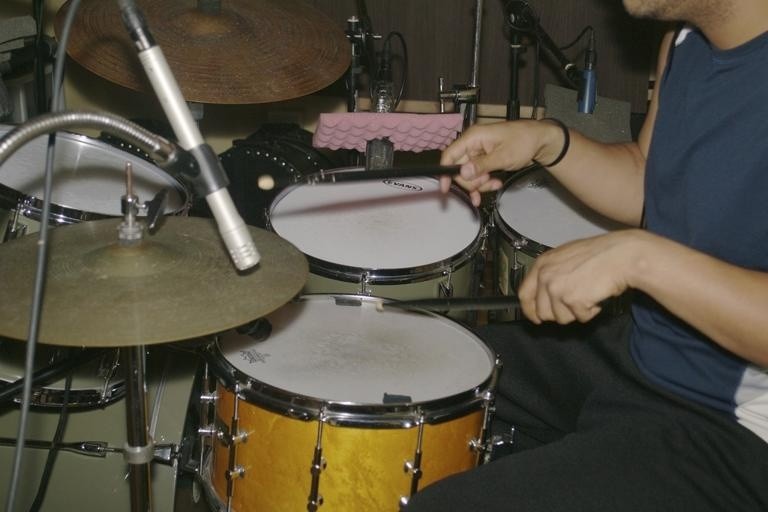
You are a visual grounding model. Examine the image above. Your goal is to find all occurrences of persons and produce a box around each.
[400,0,767,512]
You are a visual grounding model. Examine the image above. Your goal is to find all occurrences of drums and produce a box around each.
[1,123,192,412]
[266,166,489,325]
[493,161,637,324]
[203,293,503,512]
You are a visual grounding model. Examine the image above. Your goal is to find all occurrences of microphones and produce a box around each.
[577,30,595,113]
[311,42,464,171]
[122,4,261,272]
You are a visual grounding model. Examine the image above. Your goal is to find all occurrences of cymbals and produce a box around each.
[54,0,352,103]
[0,215,308,348]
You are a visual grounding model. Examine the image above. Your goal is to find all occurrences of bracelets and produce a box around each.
[531,117,569,168]
